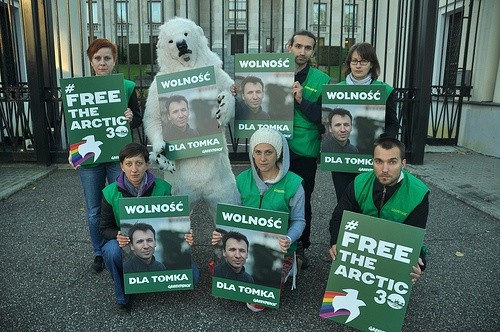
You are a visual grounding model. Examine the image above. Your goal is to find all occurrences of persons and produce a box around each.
[211,126,307,314]
[213,229,255,282]
[100,143,201,315]
[321,105,360,155]
[231,31,333,269]
[234,75,271,123]
[329,137,432,283]
[120,223,173,274]
[59,38,143,274]
[331,42,400,211]
[158,94,203,143]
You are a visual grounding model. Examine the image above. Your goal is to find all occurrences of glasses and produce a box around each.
[349,59,371,66]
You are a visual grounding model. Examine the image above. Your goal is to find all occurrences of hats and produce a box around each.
[249,127,283,161]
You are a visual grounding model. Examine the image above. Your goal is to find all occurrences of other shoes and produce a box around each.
[296,241,308,269]
[90,256,105,273]
[209,260,215,273]
[118,302,130,312]
[246,302,265,311]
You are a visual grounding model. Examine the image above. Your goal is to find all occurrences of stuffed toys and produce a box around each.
[143,19,243,226]
